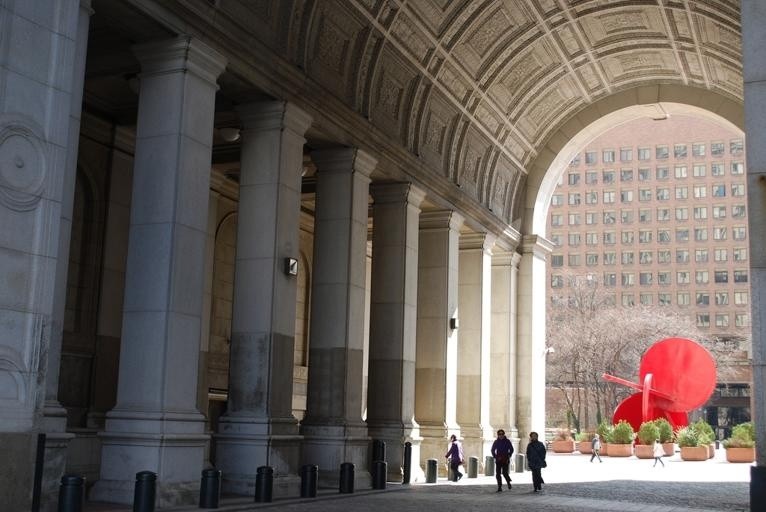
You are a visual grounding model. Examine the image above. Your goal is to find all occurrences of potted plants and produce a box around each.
[548,415,758,466]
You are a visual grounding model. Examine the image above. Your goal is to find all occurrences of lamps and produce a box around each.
[215,124,241,143]
[282,256,299,277]
[449,316,460,330]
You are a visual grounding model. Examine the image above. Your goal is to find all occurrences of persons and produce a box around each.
[652,438,666,467]
[491,429,514,493]
[588,433,604,463]
[445,435,464,482]
[526,433,546,492]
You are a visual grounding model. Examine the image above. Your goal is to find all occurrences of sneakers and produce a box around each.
[452,472,464,482]
[495,479,512,493]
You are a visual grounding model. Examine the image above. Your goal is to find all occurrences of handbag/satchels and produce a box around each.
[542,461,547,468]
